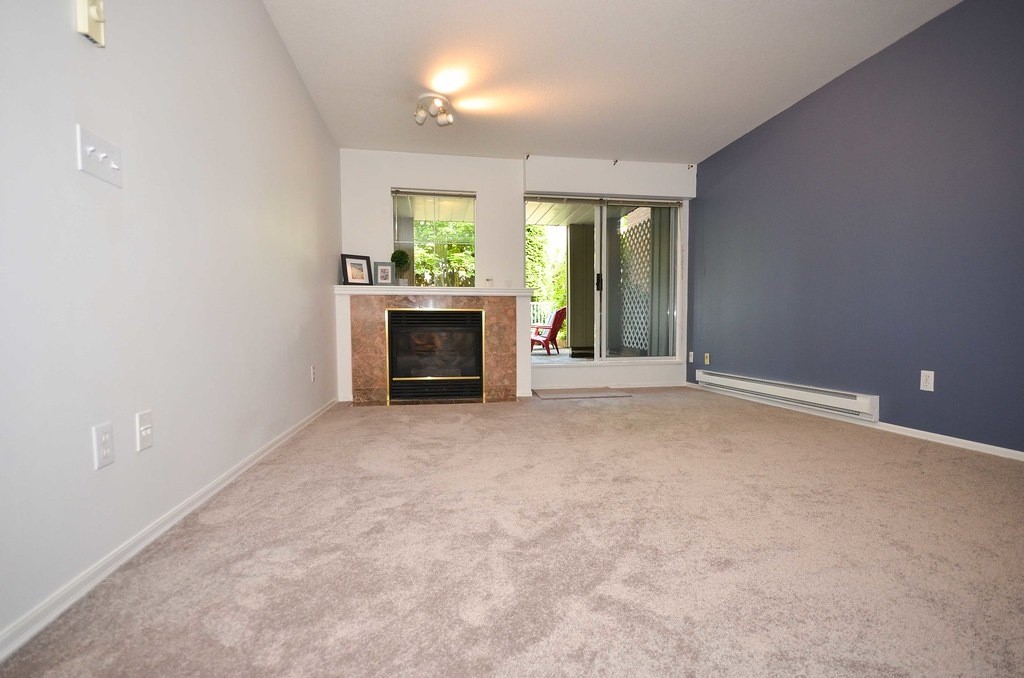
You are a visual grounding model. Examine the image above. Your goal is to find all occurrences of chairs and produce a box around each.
[531,306,567,355]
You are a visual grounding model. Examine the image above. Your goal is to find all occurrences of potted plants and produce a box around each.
[392,250,409,286]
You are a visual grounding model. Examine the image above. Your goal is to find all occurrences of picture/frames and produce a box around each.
[341,254,373,285]
[374,261,396,286]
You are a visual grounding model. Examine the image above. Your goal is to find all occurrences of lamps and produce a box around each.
[414,93,454,126]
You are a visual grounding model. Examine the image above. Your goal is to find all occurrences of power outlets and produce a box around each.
[92,422,115,471]
[136,410,153,452]
[311,365,315,383]
[689,352,694,362]
[919,370,934,392]
[705,352,710,365]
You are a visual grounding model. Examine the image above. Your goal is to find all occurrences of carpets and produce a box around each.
[531,386,633,400]
